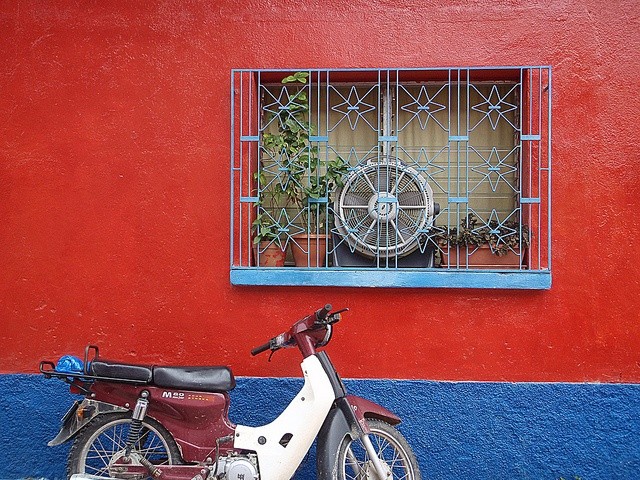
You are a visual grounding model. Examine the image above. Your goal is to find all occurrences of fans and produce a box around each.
[332,159,437,266]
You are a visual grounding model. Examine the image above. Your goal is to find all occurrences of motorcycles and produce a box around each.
[40,304,422,480]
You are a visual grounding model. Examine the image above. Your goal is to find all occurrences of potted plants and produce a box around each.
[249,130,289,266]
[267,71,348,265]
[421,213,534,268]
